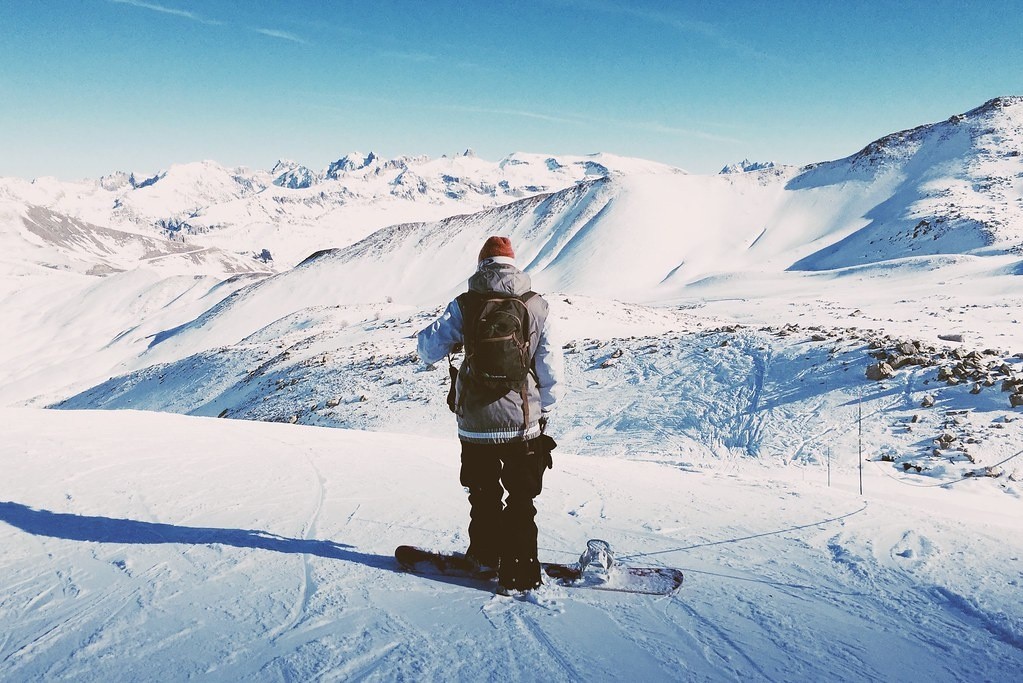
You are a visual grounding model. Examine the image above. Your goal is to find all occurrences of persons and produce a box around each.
[416,231,560,584]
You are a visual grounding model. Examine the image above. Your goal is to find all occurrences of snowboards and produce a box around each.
[395,544,684,596]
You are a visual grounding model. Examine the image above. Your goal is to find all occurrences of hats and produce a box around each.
[478,235,515,264]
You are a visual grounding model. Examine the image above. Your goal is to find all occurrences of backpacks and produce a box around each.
[460,291,540,382]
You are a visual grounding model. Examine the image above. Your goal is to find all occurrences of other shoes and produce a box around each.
[467,545,500,572]
[498,561,543,594]
